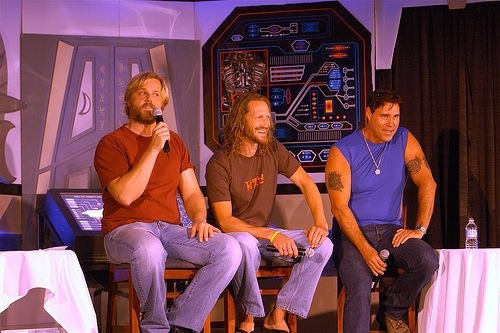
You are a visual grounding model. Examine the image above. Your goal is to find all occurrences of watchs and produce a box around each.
[415,225,426,234]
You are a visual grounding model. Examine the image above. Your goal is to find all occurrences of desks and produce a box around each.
[0,250,99,333]
[418,248,500,333]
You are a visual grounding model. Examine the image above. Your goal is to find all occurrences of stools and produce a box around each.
[220,257,299,333]
[113,257,211,333]
[336,258,418,332]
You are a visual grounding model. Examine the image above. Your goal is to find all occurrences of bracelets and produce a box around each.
[270,231,279,243]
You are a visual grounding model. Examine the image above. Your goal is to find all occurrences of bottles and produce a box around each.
[465,218,478,249]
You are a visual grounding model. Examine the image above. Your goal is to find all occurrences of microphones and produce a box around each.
[371,250,389,288]
[266,245,314,258]
[152,108,170,152]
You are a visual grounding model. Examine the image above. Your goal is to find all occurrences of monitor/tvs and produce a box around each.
[51,188,194,235]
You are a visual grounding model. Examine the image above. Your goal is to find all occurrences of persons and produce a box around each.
[325,91,439,333]
[94,71,242,333]
[206,92,334,333]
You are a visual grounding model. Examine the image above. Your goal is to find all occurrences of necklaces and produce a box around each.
[363,128,389,175]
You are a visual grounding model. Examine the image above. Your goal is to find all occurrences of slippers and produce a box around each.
[263,326,291,333]
[236,324,256,332]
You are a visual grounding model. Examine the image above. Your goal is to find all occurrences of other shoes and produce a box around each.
[378,312,411,333]
[168,325,194,333]
